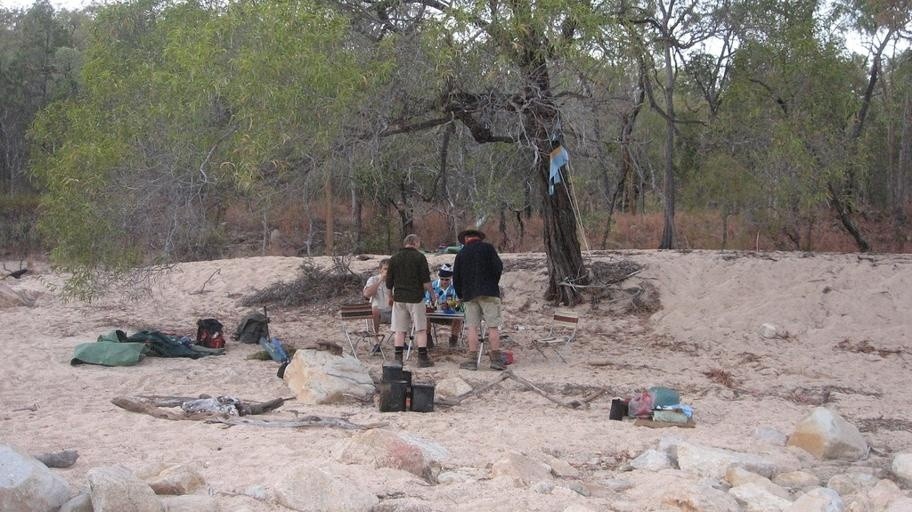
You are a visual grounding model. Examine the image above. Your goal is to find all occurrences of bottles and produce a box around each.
[260,337,288,361]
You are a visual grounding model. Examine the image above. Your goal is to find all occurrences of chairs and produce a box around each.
[533,309,578,360]
[338,302,386,363]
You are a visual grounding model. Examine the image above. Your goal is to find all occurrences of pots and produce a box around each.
[381,364,403,381]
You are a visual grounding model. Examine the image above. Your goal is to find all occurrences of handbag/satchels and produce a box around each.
[197,318,225,348]
[238,311,269,343]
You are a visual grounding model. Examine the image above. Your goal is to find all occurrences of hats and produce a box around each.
[438,263,453,277]
[458,225,485,244]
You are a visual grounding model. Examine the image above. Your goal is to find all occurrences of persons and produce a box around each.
[385,234,436,367]
[363,259,412,352]
[452,230,507,370]
[425,264,463,348]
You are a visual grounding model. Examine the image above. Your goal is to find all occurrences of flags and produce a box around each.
[549,140,568,195]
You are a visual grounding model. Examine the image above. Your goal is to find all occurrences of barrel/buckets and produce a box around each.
[378,361,436,414]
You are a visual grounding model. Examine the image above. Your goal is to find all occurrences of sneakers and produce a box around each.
[460,361,478,370]
[448,344,457,352]
[374,344,381,352]
[490,360,506,370]
[404,342,412,350]
[428,342,434,349]
[418,358,434,367]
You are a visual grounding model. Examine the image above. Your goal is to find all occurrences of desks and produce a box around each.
[404,311,494,368]
